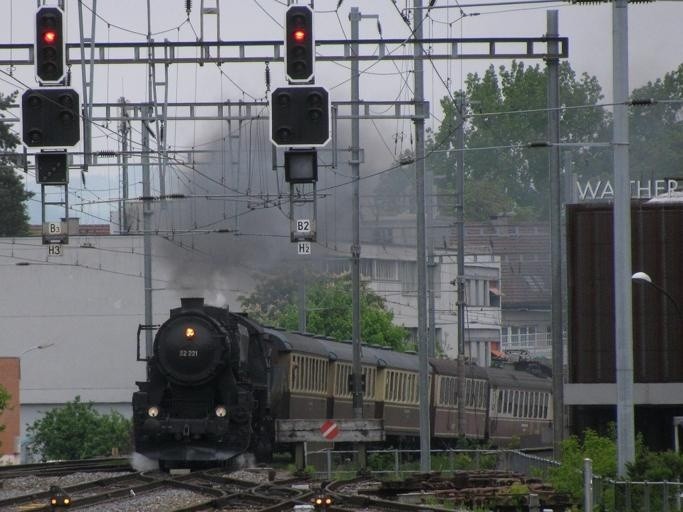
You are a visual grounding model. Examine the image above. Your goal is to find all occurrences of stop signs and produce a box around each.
[320,421,338,439]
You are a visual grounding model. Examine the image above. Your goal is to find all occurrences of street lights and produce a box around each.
[632,271,683,319]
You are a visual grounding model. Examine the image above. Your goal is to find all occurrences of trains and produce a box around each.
[132,298,570,472]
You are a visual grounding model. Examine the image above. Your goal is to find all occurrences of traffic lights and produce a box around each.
[269,5,333,183]
[21,5,81,184]
